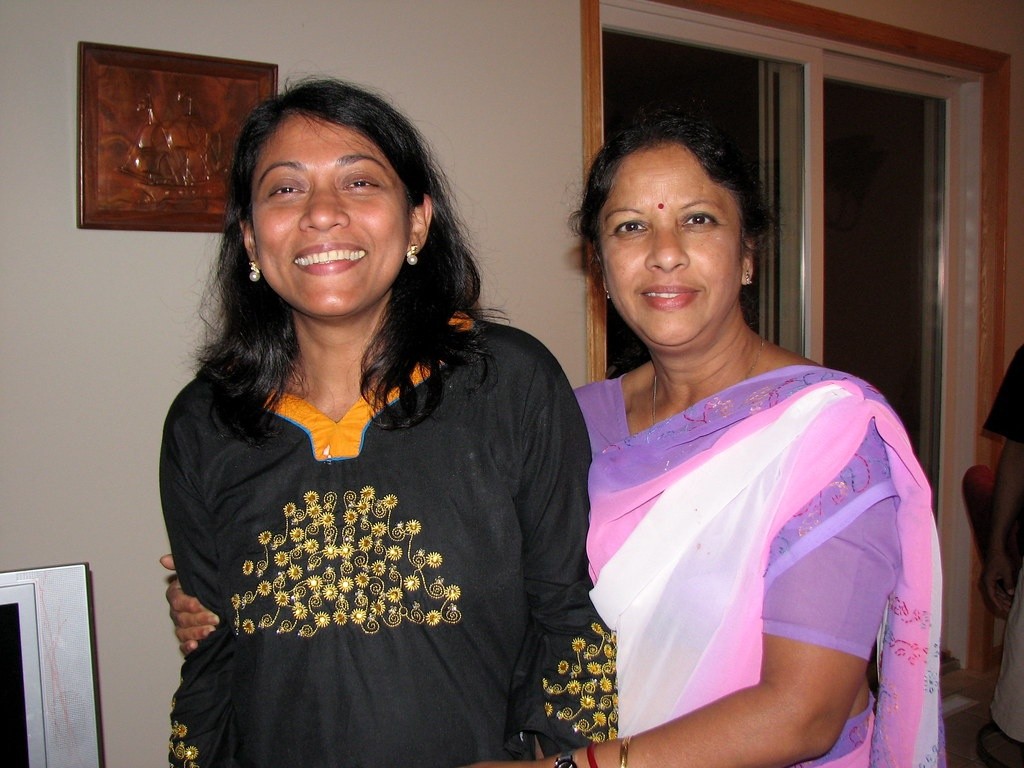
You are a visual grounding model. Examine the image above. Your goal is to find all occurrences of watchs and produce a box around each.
[554,749,577,768]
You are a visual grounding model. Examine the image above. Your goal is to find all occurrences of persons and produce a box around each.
[982,343,1024,768]
[160,76,619,768]
[161,104,943,768]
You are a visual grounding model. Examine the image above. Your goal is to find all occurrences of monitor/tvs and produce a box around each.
[0,563,107,768]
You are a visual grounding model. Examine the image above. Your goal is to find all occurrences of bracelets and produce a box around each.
[620,735,632,768]
[587,742,599,768]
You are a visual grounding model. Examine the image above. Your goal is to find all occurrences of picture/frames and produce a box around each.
[75,41,278,231]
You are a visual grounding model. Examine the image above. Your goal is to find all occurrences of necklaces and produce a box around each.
[652,337,764,425]
